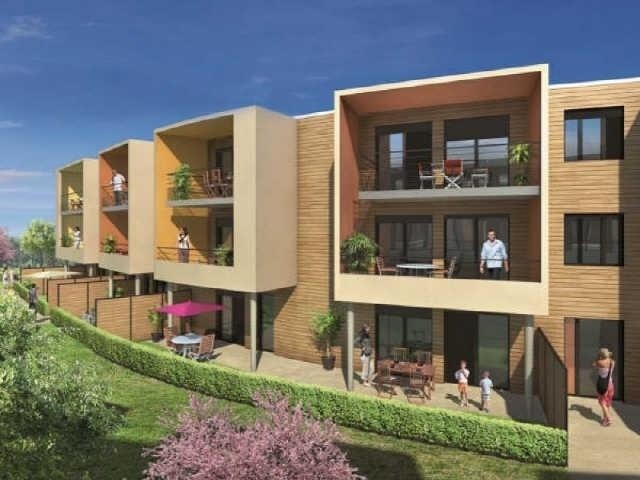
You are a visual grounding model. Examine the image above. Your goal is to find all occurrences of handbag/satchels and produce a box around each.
[597,376,608,395]
